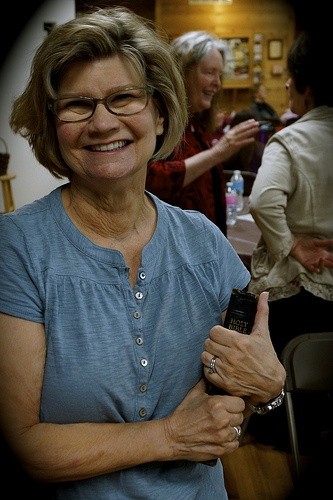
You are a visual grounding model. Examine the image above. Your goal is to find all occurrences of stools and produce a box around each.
[0,172,17,213]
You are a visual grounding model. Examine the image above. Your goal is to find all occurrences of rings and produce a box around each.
[208,355,219,375]
[234,425,242,441]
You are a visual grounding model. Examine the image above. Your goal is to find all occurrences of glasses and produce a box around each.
[48,87,154,123]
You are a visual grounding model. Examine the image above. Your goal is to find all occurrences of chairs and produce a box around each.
[280,332,333,500]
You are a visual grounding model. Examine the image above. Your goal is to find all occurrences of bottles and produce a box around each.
[230,170,244,211]
[224,181,238,226]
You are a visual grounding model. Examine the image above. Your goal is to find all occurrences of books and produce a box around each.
[200,288,260,467]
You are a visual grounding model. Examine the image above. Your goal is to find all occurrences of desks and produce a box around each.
[225,197,263,267]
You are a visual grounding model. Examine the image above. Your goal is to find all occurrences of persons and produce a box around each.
[246,29,333,456]
[222,84,277,171]
[0,6,288,500]
[145,30,260,239]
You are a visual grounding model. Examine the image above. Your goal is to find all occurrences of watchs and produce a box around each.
[244,387,285,415]
